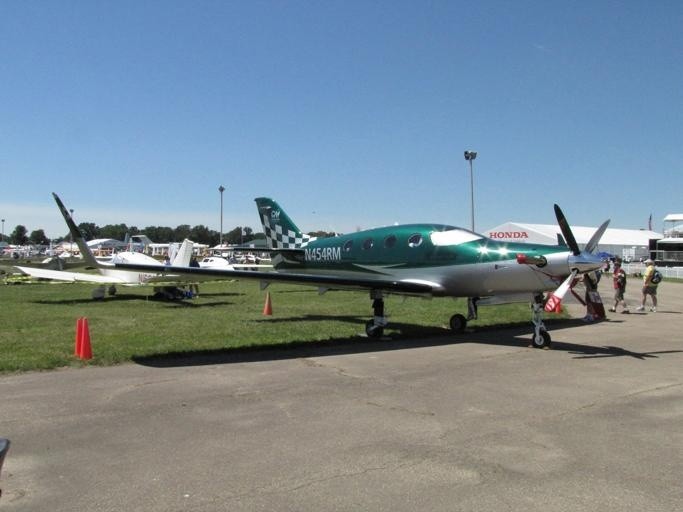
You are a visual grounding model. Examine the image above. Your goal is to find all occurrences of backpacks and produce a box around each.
[649,266,662,284]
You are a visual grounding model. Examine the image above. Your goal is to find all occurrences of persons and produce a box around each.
[636,259,658,313]
[607,261,629,314]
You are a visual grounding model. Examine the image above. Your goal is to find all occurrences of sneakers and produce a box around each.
[635,307,645,311]
[649,307,656,312]
[608,309,616,312]
[620,309,629,314]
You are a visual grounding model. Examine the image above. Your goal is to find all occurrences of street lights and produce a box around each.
[218,186,224,257]
[69,209,73,256]
[463,149,477,233]
[1,219,5,241]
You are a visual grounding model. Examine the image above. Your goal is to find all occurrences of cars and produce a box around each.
[234,255,256,263]
[596,251,616,261]
[197,257,229,268]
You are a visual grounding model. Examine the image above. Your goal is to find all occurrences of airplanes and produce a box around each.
[114,196,612,348]
[12,191,240,302]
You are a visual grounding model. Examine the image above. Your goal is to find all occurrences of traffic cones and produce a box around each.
[262,291,273,316]
[79,317,92,360]
[74,318,83,357]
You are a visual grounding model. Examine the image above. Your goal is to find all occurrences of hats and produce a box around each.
[643,259,654,264]
